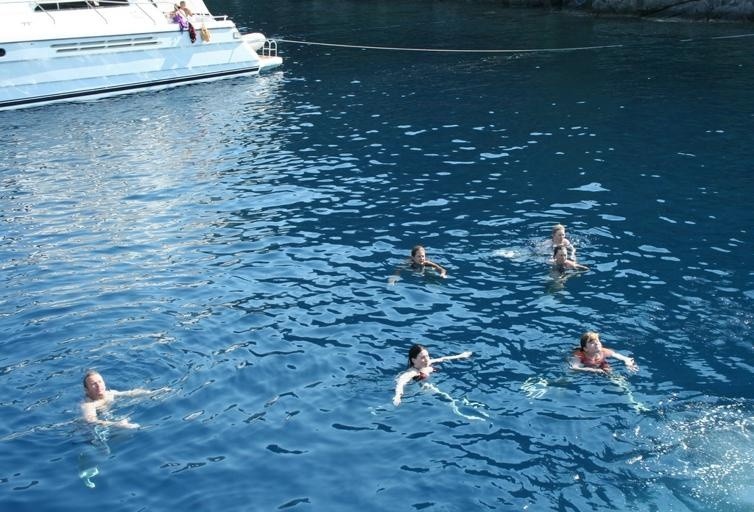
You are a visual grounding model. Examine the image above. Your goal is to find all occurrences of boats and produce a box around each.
[0,1,282,110]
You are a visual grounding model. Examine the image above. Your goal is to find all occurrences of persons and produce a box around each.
[547,245,588,290]
[78,370,171,489]
[539,223,577,260]
[171,1,192,21]
[391,344,489,422]
[569,332,648,415]
[387,245,447,283]
